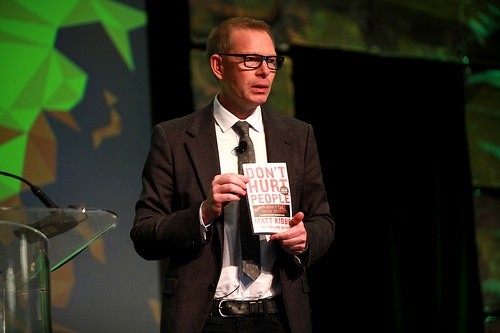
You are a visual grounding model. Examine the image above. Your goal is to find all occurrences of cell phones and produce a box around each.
[14,214,79,243]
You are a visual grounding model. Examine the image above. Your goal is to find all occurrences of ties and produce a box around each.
[231,121,261,281]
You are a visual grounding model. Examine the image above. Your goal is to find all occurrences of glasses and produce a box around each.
[217,53,285,70]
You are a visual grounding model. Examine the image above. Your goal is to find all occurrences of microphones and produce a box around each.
[0,172,59,208]
[234,141,248,154]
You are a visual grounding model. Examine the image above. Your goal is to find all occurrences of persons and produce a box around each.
[130,16,336,333]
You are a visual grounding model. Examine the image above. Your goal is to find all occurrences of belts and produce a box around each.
[211,299,283,318]
